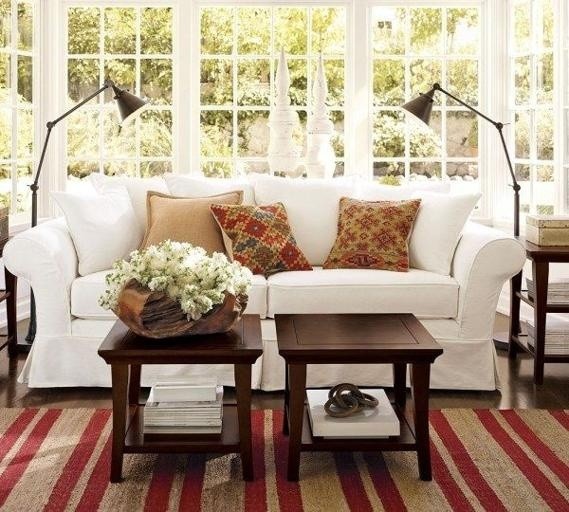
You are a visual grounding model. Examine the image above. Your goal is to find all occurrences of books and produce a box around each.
[526,278,569,304]
[306,389,400,439]
[527,319,569,355]
[143,382,223,434]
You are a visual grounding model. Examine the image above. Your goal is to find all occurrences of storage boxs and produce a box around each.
[524,211,569,246]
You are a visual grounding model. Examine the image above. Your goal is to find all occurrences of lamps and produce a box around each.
[17,78,151,356]
[400,79,521,352]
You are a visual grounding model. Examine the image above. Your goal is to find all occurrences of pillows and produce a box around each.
[248,166,345,268]
[138,187,245,259]
[207,197,316,276]
[120,174,171,243]
[45,180,145,276]
[319,194,424,272]
[406,190,482,278]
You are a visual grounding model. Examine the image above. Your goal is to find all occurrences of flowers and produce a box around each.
[98,239,257,320]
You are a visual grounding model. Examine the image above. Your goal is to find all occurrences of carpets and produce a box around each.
[0,404,568,512]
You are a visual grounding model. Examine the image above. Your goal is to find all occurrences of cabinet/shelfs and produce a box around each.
[0,236,20,371]
[506,237,568,386]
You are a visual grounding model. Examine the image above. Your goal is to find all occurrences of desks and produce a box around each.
[272,310,446,486]
[98,311,266,483]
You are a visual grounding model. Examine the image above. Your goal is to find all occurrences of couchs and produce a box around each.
[0,174,527,392]
